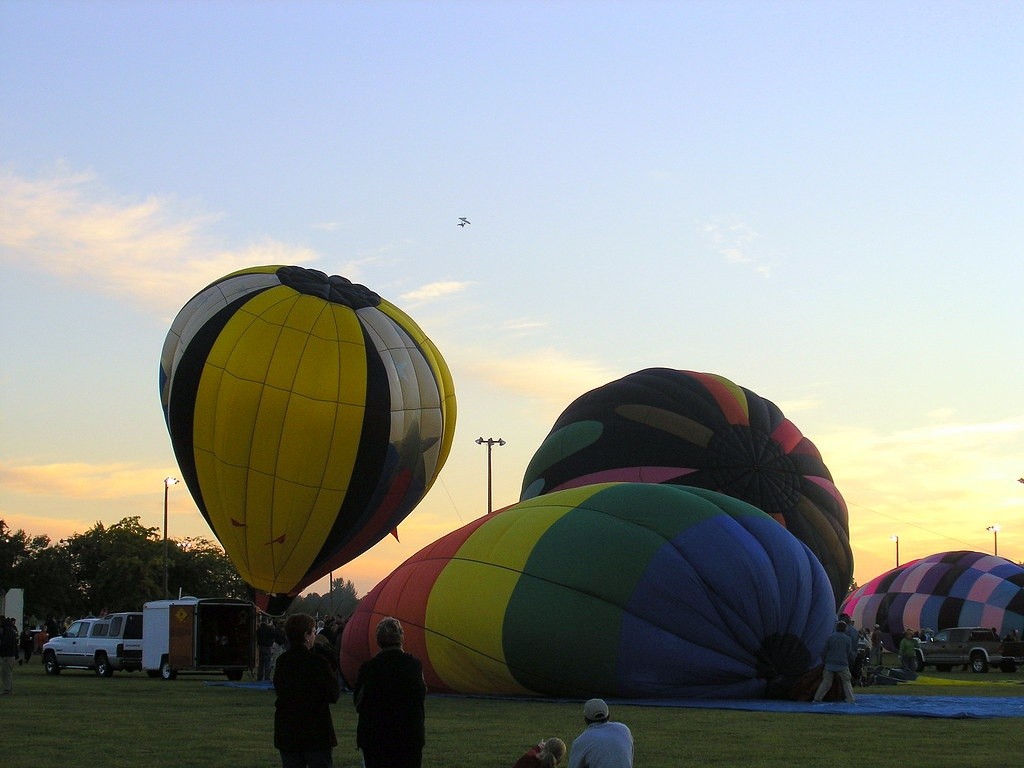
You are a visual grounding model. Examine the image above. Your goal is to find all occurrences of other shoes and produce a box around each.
[0,691,10,696]
[19,660,22,665]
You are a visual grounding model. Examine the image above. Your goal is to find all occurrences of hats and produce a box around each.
[584,699,608,720]
[874,624,879,628]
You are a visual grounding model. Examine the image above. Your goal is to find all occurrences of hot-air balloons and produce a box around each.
[155,264,458,667]
[342,367,1024,706]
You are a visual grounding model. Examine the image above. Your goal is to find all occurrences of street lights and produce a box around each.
[986,525,997,556]
[163,476,181,599]
[475,437,507,514]
[890,536,898,567]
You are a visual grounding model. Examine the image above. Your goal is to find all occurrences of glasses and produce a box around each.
[312,630,317,635]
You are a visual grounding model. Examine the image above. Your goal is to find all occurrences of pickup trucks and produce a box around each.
[42,612,143,677]
[915,627,1024,674]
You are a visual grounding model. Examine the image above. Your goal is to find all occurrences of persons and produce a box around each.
[990,624,1018,641]
[513,737,566,768]
[0,608,113,661]
[0,619,18,696]
[566,698,635,768]
[256,606,347,684]
[352,617,427,768]
[835,612,860,673]
[858,624,935,668]
[812,621,858,705]
[273,614,342,768]
